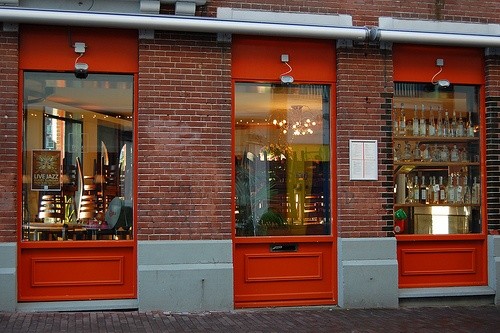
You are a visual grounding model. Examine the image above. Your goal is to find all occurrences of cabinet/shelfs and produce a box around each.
[394,134,479,207]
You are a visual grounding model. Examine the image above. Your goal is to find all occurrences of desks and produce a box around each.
[22,222,88,240]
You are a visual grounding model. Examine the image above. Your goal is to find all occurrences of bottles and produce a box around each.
[394,140,469,163]
[398,103,407,136]
[437,107,443,136]
[420,104,426,136]
[466,111,474,136]
[429,105,436,136]
[443,112,450,136]
[392,102,398,136]
[408,174,481,205]
[450,110,465,137]
[413,104,418,135]
